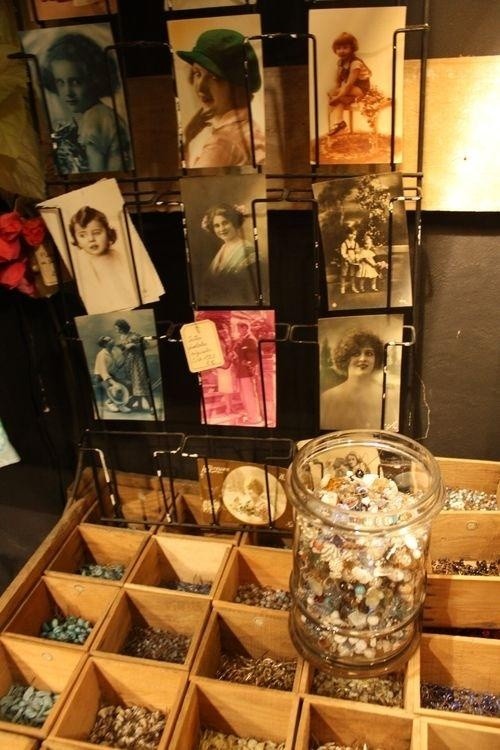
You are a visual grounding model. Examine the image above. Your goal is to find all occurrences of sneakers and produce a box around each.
[105,401,131,413]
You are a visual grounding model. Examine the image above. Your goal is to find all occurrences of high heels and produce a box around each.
[149,405,155,415]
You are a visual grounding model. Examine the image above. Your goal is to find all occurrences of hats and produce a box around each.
[175,29,261,93]
[55,120,70,132]
[106,383,129,405]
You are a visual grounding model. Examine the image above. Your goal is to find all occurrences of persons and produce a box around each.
[201,204,268,304]
[340,227,379,294]
[176,30,266,169]
[320,329,399,429]
[42,33,132,172]
[214,323,264,424]
[70,205,159,315]
[327,33,372,136]
[94,320,155,415]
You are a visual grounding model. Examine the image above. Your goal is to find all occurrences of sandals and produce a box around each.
[327,121,347,137]
